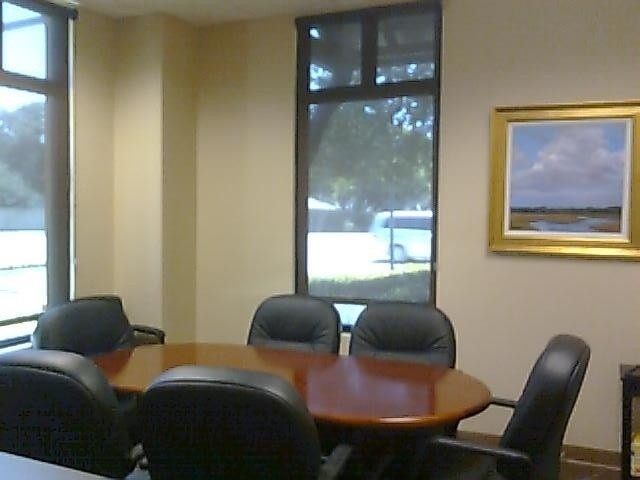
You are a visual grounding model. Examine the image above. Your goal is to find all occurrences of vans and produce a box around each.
[365,208,433,264]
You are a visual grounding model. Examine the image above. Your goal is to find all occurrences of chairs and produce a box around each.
[0,348,149,480]
[31,291,167,358]
[245,293,341,355]
[415,330,592,479]
[346,300,456,369]
[138,363,365,480]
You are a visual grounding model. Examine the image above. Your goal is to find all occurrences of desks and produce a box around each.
[91,340,492,480]
[619,362,639,479]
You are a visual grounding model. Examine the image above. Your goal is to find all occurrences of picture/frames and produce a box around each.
[486,97,640,262]
